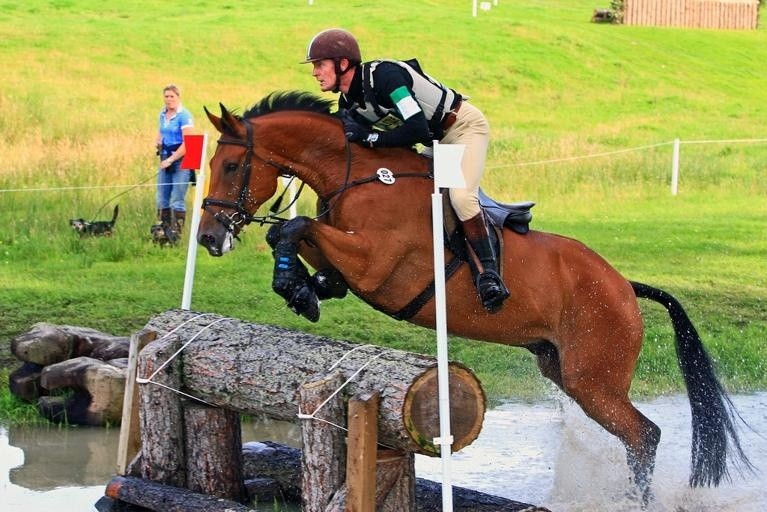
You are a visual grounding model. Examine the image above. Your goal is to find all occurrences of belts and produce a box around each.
[446,101,462,129]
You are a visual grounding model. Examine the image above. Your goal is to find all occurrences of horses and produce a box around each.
[195,87,763,512]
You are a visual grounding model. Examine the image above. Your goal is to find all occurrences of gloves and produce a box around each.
[344,121,381,149]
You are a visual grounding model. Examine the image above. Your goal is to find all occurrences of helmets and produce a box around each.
[298,28,362,64]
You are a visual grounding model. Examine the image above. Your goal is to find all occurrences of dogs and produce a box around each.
[69,204,119,239]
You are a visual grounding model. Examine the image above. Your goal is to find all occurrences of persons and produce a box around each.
[298,28,501,304]
[153,84,197,244]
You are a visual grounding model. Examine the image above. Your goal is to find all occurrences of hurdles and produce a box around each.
[93,173,484,512]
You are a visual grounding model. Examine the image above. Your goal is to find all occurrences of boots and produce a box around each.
[460,211,503,315]
[173,208,186,238]
[158,209,171,224]
